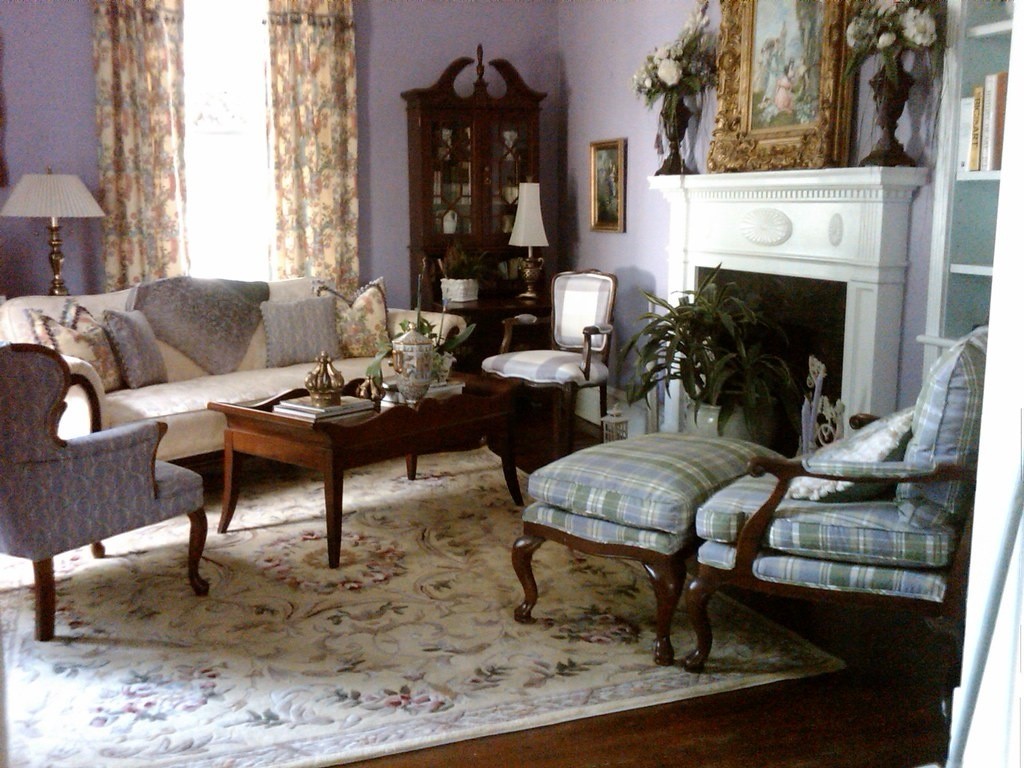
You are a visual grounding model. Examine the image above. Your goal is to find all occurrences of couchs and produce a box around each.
[1,277,466,460]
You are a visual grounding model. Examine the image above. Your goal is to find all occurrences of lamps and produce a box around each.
[507,183,549,301]
[1,166,104,296]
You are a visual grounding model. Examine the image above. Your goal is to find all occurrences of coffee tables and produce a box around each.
[207,365,527,570]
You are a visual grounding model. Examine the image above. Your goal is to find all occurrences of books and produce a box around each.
[957,71,1008,172]
[273,396,375,423]
[381,375,465,403]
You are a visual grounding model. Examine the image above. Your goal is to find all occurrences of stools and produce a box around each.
[507,435,783,666]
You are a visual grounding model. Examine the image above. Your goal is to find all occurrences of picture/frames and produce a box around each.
[587,137,628,235]
[706,0,853,172]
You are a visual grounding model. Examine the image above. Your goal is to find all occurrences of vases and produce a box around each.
[863,74,918,168]
[652,107,697,174]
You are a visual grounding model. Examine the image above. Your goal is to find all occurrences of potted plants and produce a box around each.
[435,248,490,301]
[631,270,803,443]
[368,272,476,385]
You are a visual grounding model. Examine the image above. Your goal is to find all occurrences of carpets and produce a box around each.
[0,427,848,767]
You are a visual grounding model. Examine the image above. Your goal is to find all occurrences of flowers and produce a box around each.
[844,0,946,72]
[632,2,718,104]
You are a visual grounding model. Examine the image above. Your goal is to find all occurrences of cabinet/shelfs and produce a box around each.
[918,0,1024,421]
[400,41,551,415]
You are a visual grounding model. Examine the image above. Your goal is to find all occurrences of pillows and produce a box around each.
[26,298,168,392]
[255,277,391,368]
[787,401,916,501]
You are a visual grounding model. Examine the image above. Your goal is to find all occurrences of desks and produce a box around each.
[427,291,560,368]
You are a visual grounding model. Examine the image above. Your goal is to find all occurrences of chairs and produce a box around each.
[480,269,616,460]
[683,325,989,672]
[0,342,210,640]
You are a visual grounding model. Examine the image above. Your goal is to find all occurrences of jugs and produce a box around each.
[392,323,434,405]
[356,375,386,402]
[442,209,458,233]
[306,351,343,405]
